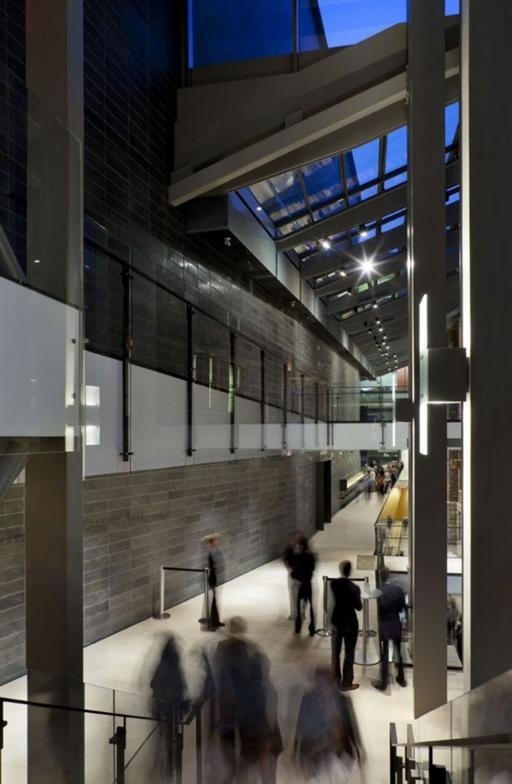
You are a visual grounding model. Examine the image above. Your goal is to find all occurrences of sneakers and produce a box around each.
[373,677,407,692]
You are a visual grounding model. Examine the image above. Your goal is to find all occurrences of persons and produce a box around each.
[327,560,363,693]
[212,614,265,784]
[195,531,225,633]
[368,566,408,693]
[282,531,307,622]
[364,458,403,498]
[149,634,193,776]
[291,534,319,638]
[243,650,286,784]
[289,661,366,784]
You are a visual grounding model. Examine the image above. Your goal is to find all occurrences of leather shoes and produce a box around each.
[339,684,359,691]
